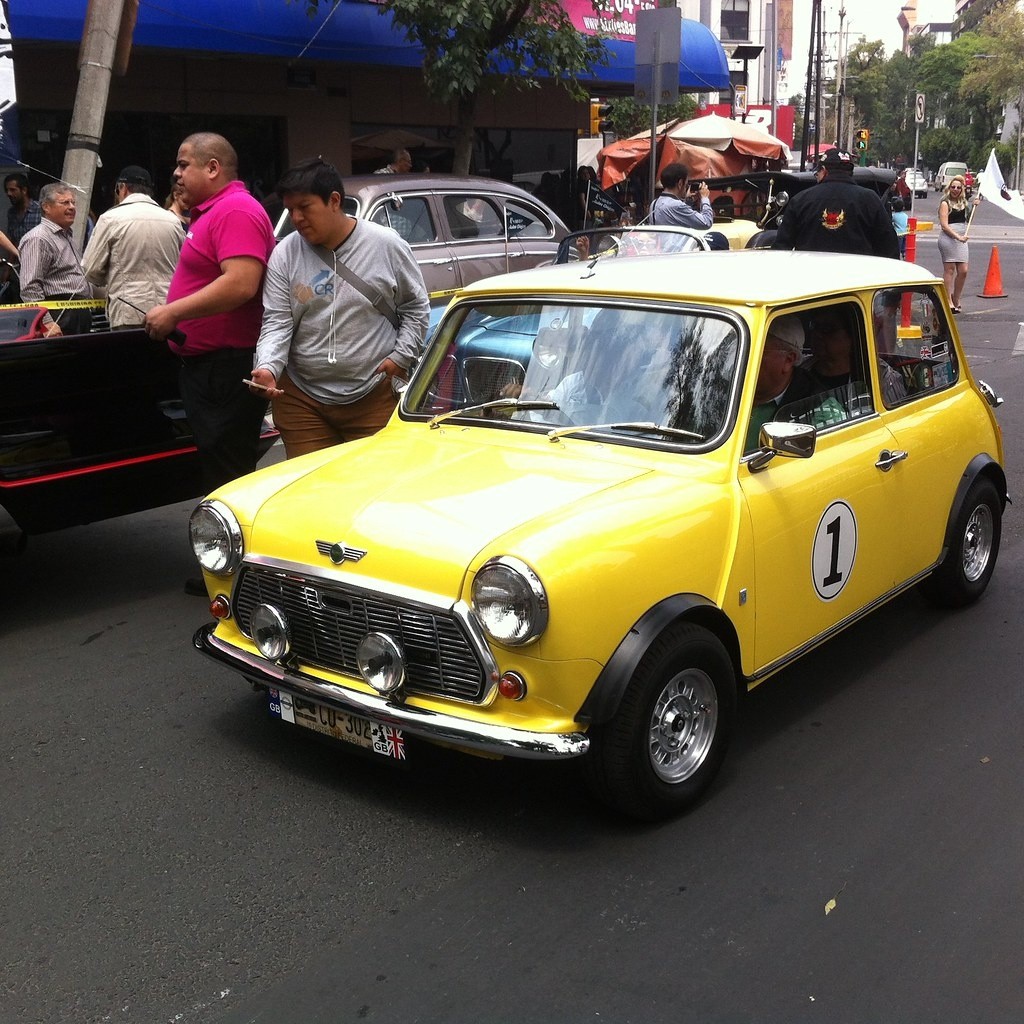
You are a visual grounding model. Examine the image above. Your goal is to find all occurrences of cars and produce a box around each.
[268,174,584,298]
[187,248,1013,814]
[417,225,712,414]
[685,171,818,250]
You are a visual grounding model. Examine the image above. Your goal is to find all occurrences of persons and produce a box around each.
[0,133,984,598]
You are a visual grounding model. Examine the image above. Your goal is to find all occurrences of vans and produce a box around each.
[935,162,968,192]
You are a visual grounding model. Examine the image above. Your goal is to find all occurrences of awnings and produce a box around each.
[1,0,730,93]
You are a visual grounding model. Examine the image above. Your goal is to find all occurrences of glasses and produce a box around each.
[951,186,961,189]
[814,169,821,179]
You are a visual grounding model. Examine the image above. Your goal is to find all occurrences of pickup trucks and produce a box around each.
[0,298,284,556]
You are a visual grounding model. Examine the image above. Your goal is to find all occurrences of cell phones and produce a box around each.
[242,379,280,392]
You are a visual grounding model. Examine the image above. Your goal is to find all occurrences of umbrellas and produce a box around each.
[596,112,794,226]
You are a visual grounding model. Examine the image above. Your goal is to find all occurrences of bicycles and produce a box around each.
[0,256,20,299]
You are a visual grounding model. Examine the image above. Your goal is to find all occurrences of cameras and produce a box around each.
[692,182,701,191]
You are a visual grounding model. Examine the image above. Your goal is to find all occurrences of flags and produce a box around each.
[978,148,1024,220]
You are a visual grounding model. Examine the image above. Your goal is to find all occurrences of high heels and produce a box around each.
[949,293,962,313]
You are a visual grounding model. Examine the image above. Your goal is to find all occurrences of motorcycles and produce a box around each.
[964,184,972,200]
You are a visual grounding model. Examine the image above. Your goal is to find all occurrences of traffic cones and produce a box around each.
[976,246,1009,299]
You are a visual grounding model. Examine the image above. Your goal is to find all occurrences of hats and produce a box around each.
[118,165,154,189]
[812,148,854,171]
[901,171,907,173]
[769,315,804,347]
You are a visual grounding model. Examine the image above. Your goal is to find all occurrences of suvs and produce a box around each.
[904,172,928,199]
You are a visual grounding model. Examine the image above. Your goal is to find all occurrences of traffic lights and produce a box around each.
[855,129,868,150]
[590,103,614,135]
